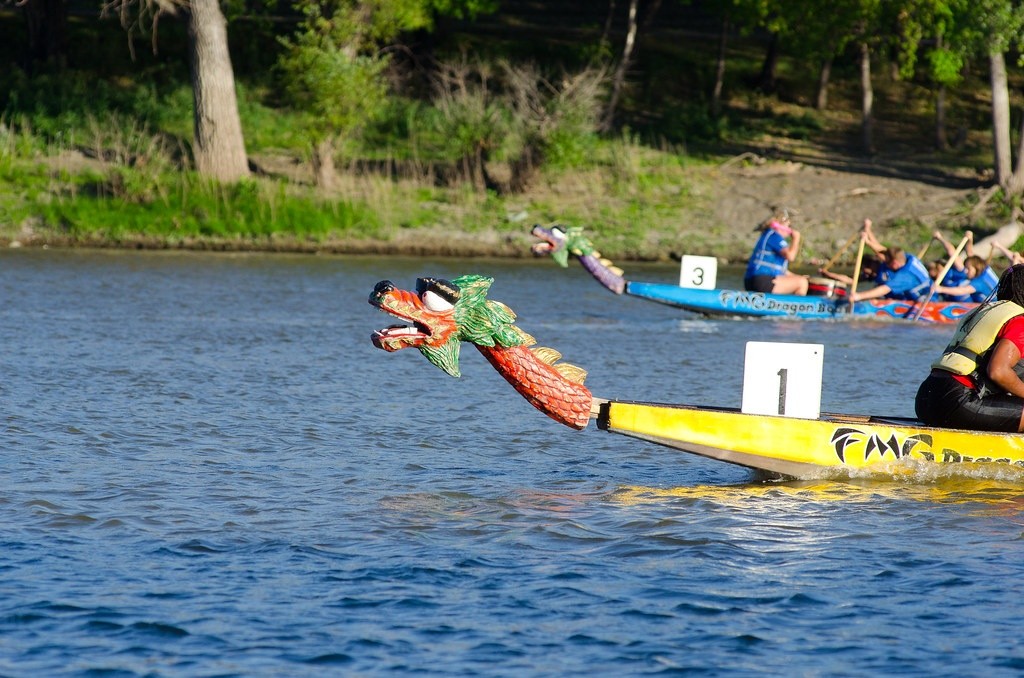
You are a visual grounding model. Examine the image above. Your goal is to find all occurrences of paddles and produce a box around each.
[914,232,936,267]
[844,224,866,318]
[818,226,865,274]
[908,236,968,324]
[985,243,998,267]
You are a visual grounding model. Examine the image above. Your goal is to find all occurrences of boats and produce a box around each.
[368,271,1024,484]
[529,221,999,329]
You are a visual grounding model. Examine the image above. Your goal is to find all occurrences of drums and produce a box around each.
[804,276,847,301]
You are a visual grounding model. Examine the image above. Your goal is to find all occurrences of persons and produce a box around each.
[744,208,809,296]
[914,263,1024,434]
[817,219,1024,303]
